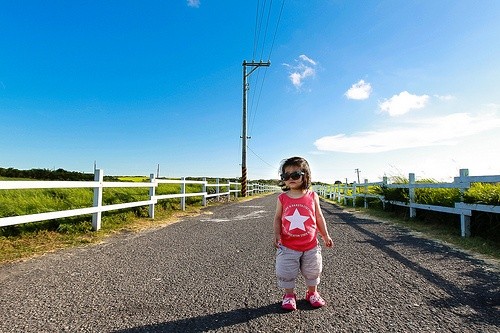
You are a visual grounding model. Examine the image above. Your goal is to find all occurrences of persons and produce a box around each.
[273,157,334,309]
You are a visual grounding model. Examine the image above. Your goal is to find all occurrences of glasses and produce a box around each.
[280,172,305,181]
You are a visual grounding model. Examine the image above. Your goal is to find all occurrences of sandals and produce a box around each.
[282,293,296,309]
[305,291,325,307]
